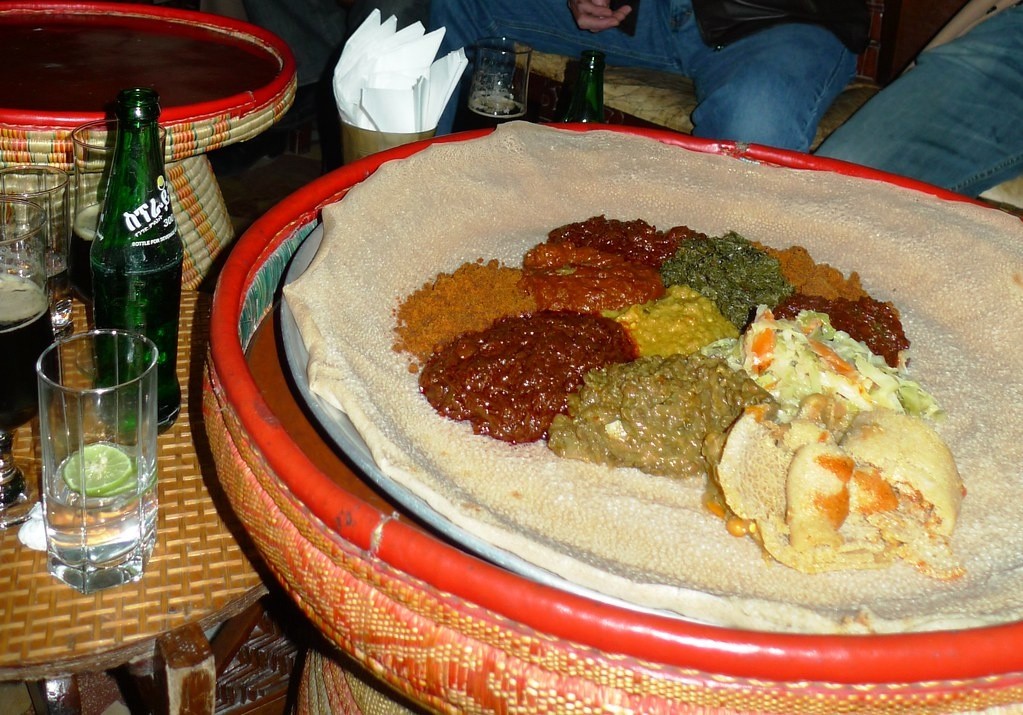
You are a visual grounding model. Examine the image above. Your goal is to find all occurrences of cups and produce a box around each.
[467,36,534,129]
[0,165,75,341]
[341,119,439,167]
[34,328,158,595]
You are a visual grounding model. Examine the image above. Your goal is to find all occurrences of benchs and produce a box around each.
[513,0,882,150]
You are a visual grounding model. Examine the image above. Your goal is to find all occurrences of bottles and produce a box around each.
[90,88,181,436]
[560,50,606,123]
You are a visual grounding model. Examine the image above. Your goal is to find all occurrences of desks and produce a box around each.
[0,289,270,715]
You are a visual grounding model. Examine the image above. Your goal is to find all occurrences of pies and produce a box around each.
[280,119,1022,635]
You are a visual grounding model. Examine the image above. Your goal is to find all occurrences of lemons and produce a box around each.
[64,444,144,497]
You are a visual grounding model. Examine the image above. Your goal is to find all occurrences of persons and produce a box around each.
[813,0,1022,198]
[241,0,430,134]
[431,0,872,151]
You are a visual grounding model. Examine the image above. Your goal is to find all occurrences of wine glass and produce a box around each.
[71,119,168,377]
[0,198,58,531]
